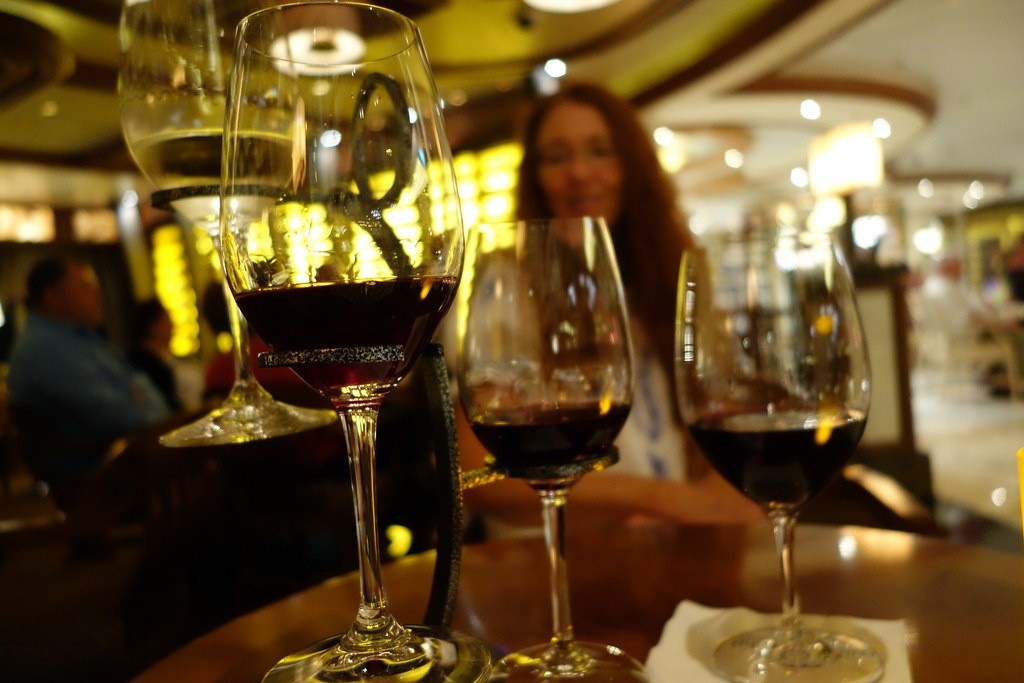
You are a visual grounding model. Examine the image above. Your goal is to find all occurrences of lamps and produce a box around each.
[808,117,886,267]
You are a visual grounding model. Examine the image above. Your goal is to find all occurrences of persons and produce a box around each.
[118,296,186,416]
[197,281,235,415]
[4,246,232,523]
[448,79,768,529]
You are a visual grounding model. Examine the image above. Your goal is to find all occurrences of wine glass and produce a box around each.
[671,230,890,683]
[216,1,492,683]
[114,0,339,450]
[445,215,650,683]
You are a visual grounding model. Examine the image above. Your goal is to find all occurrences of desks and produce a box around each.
[132,520,1024,683]
